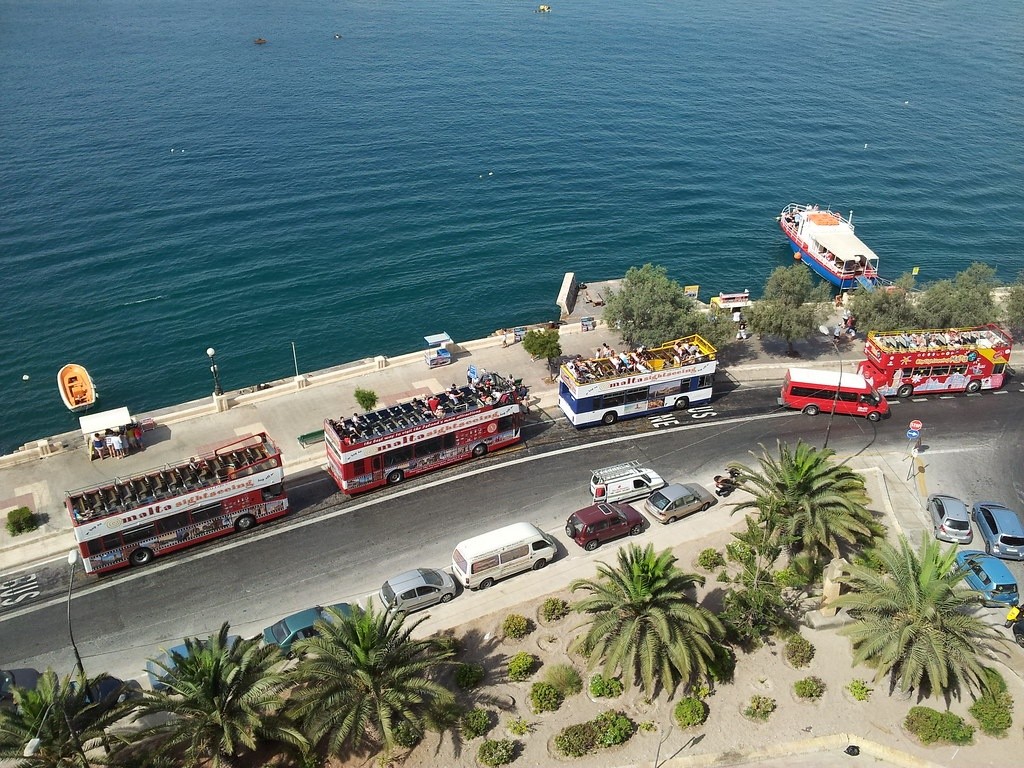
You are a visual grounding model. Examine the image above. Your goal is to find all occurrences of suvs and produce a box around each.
[565,502,645,552]
[589,460,668,507]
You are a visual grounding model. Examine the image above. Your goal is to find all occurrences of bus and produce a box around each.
[62,430,295,575]
[323,372,522,496]
[853,321,1014,399]
[555,334,720,430]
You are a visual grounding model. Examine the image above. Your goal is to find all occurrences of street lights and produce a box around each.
[206,348,223,395]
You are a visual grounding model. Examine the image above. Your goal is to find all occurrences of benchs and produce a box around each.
[881,331,1007,351]
[585,288,604,307]
[72,442,273,523]
[564,342,714,386]
[327,386,486,447]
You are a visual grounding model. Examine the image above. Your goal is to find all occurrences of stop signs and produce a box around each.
[910,420,922,430]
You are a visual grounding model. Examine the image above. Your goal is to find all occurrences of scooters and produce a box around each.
[713,467,744,497]
[1004,603,1024,628]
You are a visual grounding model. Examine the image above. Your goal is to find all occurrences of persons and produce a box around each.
[839,300,861,340]
[73,507,89,519]
[189,456,202,472]
[831,322,843,346]
[75,511,94,523]
[501,329,509,348]
[326,365,531,450]
[735,318,748,342]
[867,325,993,358]
[568,333,705,389]
[780,202,818,230]
[92,422,146,462]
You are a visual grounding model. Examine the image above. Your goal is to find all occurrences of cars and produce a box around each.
[52,677,127,718]
[1012,620,1024,650]
[263,602,368,660]
[925,492,973,546]
[971,501,1024,561]
[953,550,1019,607]
[143,635,248,694]
[643,482,716,525]
[378,567,457,623]
[0,666,43,704]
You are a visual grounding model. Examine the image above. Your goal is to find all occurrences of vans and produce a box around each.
[776,367,892,422]
[451,520,558,592]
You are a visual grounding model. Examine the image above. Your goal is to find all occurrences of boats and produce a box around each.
[775,202,880,297]
[57,363,100,413]
